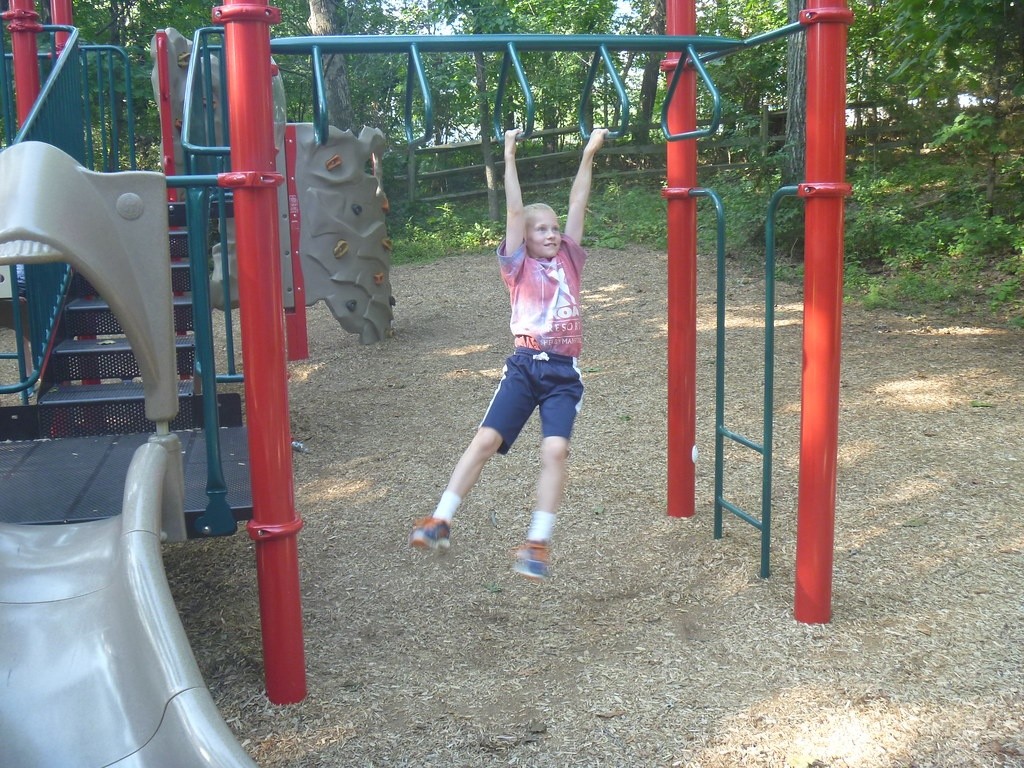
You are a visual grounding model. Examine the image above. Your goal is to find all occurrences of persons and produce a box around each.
[409,129,611,580]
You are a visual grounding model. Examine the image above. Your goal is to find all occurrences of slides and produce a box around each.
[0,433,261,768]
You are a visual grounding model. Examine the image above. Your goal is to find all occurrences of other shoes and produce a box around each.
[518,541,548,580]
[409,519,450,550]
